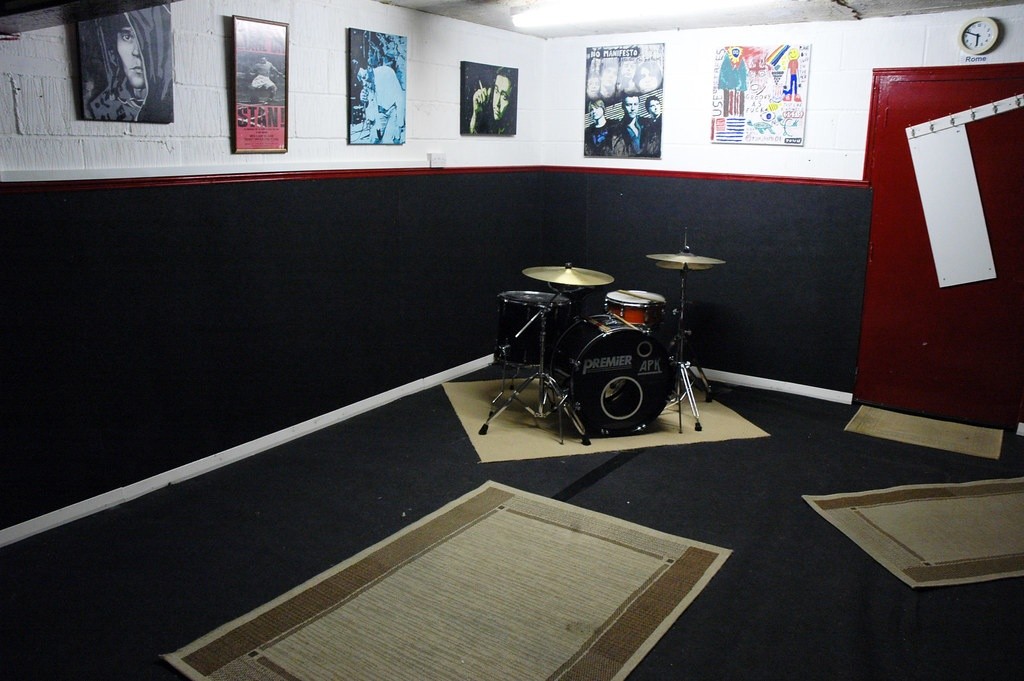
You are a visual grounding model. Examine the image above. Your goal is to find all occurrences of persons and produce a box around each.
[249,56,282,102]
[354,61,406,144]
[584,59,662,157]
[470,66,518,134]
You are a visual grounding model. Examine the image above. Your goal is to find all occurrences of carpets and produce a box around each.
[441,377,773,464]
[159,480,734,681]
[801,477,1024,589]
[843,405,1005,460]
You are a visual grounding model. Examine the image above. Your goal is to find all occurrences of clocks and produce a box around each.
[957,11,1001,58]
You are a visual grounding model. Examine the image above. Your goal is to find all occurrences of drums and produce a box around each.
[604,289,668,327]
[491,289,571,370]
[546,312,674,437]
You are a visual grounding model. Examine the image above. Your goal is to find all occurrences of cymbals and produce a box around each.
[521,266,614,285]
[646,253,727,270]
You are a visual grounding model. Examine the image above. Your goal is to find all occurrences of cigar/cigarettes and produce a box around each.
[479,80,482,89]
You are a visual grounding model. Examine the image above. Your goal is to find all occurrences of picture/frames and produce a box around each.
[231,14,290,153]
